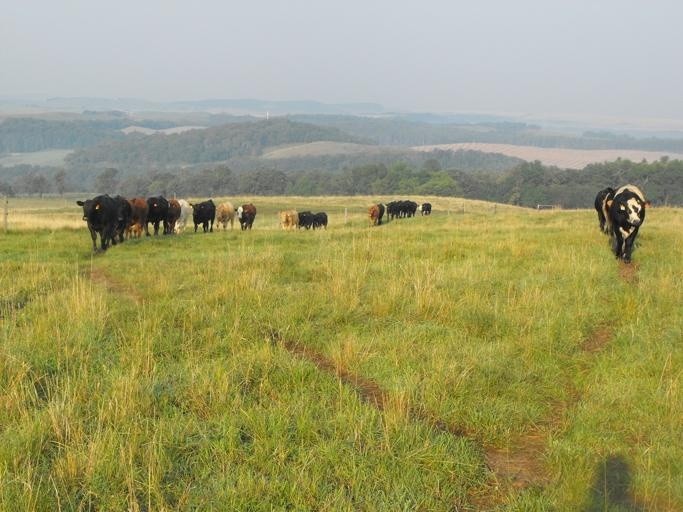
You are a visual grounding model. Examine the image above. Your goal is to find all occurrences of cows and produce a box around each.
[591,182,650,265]
[75,192,235,251]
[235,201,257,231]
[366,200,432,226]
[278,207,328,230]
